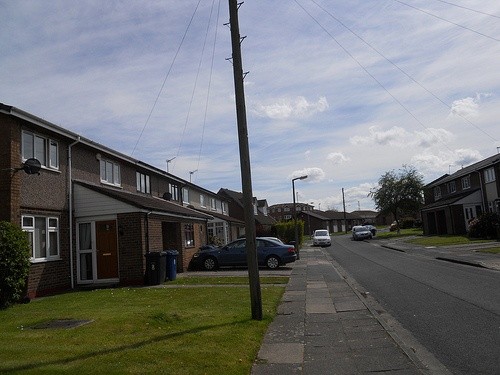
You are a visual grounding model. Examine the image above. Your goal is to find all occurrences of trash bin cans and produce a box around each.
[144,251,168,285]
[163,249,179,281]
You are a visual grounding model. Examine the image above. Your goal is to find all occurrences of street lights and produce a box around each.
[292,175,308,260]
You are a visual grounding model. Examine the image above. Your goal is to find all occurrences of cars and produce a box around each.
[311,230,332,246]
[193,237,298,270]
[391,220,399,227]
[351,224,377,240]
